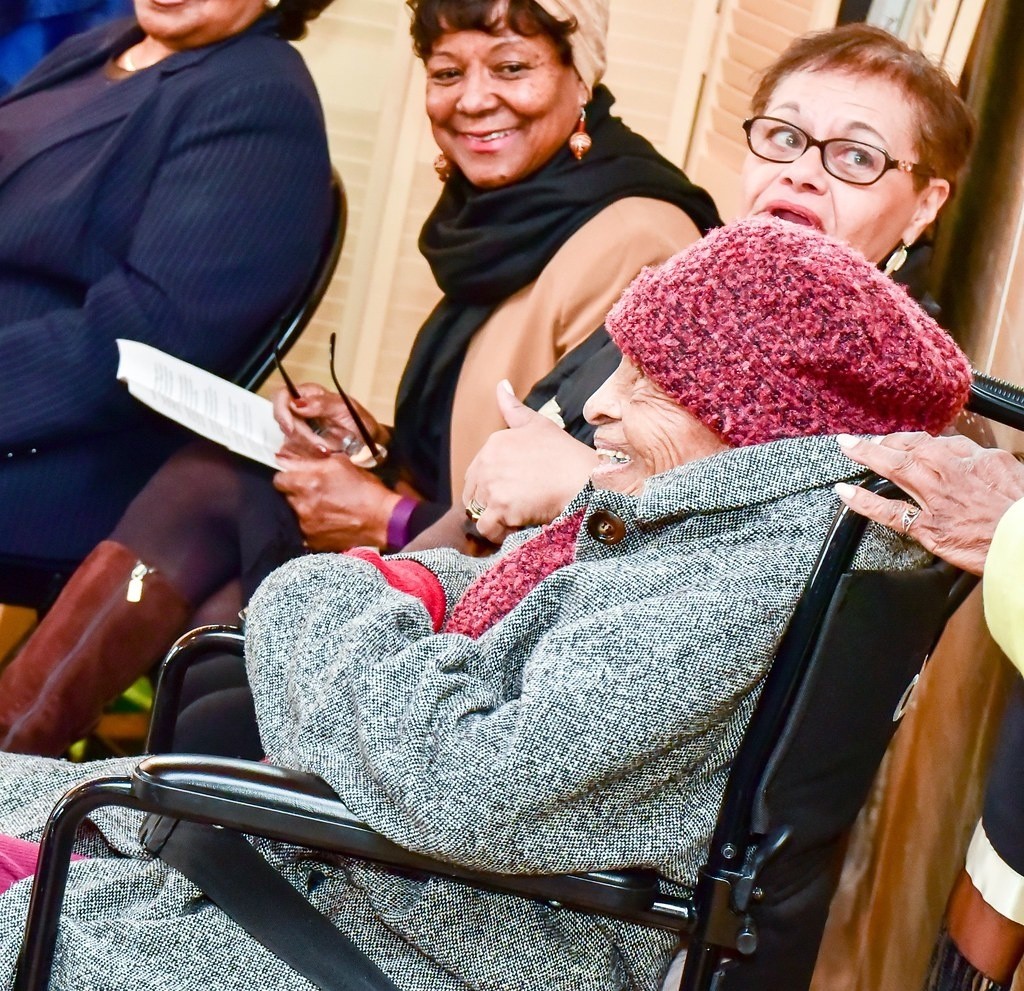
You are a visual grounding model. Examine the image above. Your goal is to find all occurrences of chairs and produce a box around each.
[12,473,980,991]
[0,176,354,664]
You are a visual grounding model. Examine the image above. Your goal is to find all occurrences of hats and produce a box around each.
[603,216,974,449]
[537,0,611,101]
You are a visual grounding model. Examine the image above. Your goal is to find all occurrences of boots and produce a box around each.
[0,540,197,761]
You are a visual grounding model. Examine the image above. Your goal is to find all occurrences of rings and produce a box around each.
[465,497,485,524]
[898,506,922,530]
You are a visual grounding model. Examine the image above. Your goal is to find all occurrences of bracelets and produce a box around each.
[384,496,420,553]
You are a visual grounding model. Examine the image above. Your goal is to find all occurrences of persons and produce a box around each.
[3,210,976,990]
[178,18,973,762]
[0,0,137,101]
[0,1,727,753]
[827,421,1024,991]
[1,0,343,613]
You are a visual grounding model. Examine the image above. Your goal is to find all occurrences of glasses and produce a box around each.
[740,114,937,185]
[273,331,390,470]
